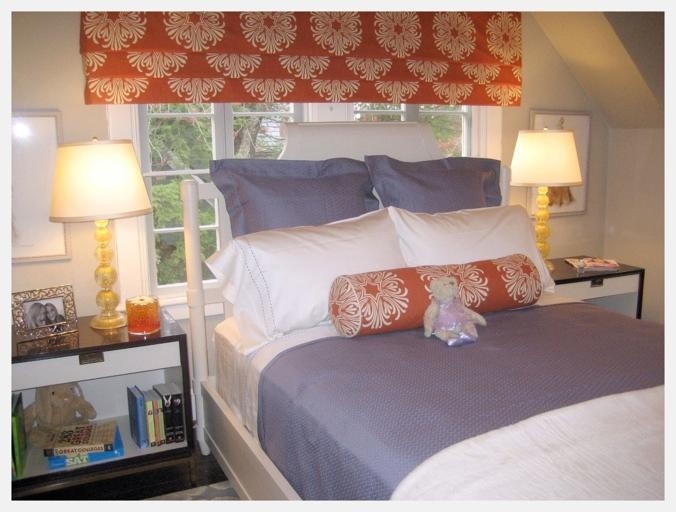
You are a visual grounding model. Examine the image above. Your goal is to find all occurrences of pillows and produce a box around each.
[499,165,511,206]
[208,155,381,241]
[361,153,505,215]
[326,251,543,341]
[202,206,408,358]
[386,205,557,296]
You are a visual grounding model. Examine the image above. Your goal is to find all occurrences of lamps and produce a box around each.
[46,135,157,333]
[509,127,585,273]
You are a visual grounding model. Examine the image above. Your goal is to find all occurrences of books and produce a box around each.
[12,391,29,479]
[565,255,621,275]
[47,426,123,469]
[126,381,185,450]
[40,420,120,456]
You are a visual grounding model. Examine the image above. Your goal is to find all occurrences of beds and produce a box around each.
[179,164,663,499]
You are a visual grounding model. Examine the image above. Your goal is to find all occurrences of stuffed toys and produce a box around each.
[422,273,487,346]
[20,381,99,450]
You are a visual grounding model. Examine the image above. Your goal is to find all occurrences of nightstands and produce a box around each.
[546,254,647,319]
[11,306,198,498]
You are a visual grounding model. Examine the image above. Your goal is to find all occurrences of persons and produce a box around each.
[24,303,48,329]
[44,302,66,324]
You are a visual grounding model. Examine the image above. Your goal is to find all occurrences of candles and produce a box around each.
[124,293,162,333]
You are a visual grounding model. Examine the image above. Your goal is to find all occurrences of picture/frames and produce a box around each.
[527,108,595,221]
[11,106,75,268]
[11,283,80,346]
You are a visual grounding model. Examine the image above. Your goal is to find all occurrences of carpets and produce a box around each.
[139,478,241,500]
